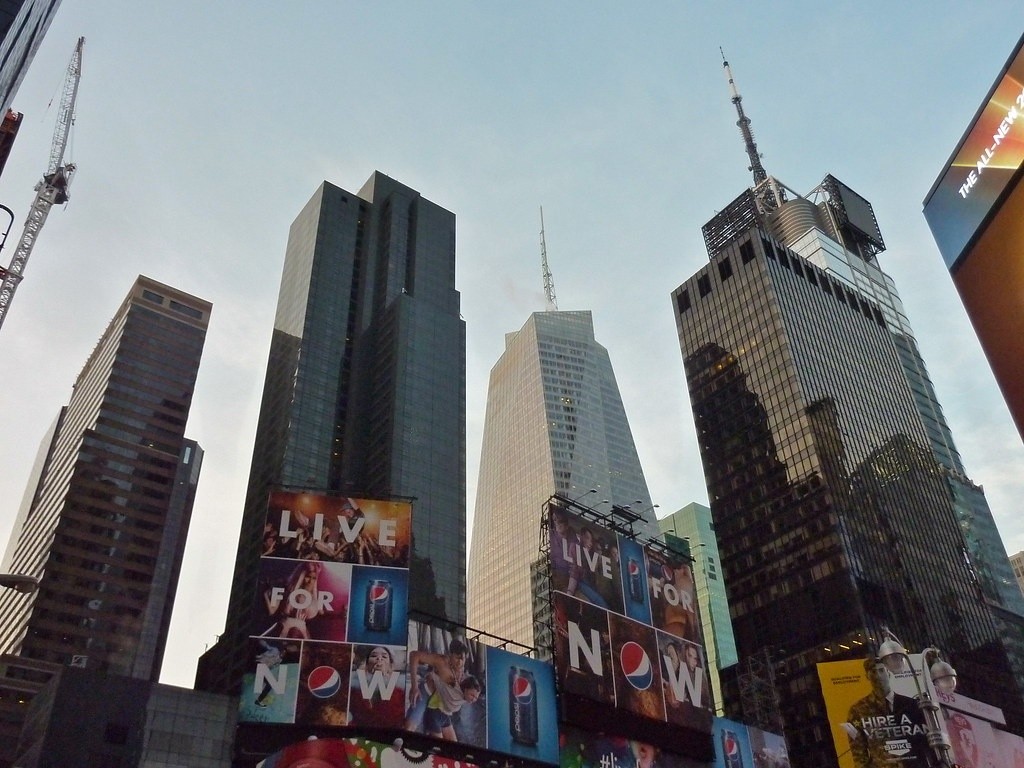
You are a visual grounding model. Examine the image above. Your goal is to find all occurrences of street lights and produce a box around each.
[878,622,960,768]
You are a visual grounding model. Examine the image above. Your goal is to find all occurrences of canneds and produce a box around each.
[508,666,538,746]
[721,728,744,768]
[627,556,644,601]
[363,577,394,632]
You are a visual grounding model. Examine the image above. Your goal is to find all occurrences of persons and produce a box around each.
[262,490,410,567]
[263,561,326,639]
[425,667,480,742]
[405,637,469,744]
[353,646,399,677]
[550,506,793,768]
[245,642,299,709]
[839,658,939,768]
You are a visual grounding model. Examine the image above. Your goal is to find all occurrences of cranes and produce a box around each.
[0,33,86,322]
